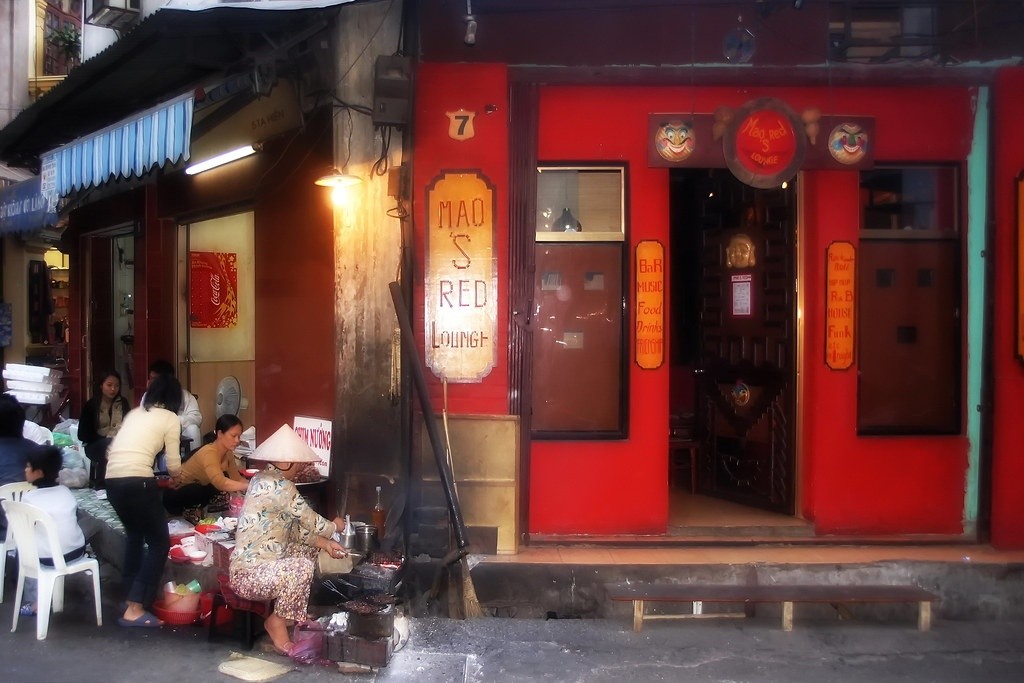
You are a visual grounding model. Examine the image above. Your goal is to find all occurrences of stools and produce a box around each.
[209,592,274,650]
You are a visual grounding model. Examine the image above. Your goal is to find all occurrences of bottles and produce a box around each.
[371,486,384,539]
[52,280,56,288]
[552,208,582,232]
[60,316,69,342]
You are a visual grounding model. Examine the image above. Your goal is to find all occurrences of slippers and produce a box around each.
[263,641,295,655]
[119,610,166,627]
[118,600,129,611]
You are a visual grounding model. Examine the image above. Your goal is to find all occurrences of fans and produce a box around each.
[215,376,249,419]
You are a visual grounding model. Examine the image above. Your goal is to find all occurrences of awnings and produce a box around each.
[0,177,61,234]
[40,82,221,198]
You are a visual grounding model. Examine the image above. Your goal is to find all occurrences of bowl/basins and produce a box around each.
[167,533,195,546]
[188,551,207,562]
[170,547,190,563]
[195,525,221,533]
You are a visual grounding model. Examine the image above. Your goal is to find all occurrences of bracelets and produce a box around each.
[332,522,337,531]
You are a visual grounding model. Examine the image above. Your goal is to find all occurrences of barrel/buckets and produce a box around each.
[163,591,202,612]
[199,589,234,628]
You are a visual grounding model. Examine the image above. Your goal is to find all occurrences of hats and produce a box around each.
[247,424,323,462]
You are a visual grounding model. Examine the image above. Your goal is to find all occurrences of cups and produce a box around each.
[52,295,69,306]
[163,581,177,593]
[340,533,356,548]
[118,293,131,307]
[185,579,202,593]
[354,525,378,550]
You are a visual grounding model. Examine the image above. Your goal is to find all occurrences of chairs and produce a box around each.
[668,368,701,495]
[1,500,102,641]
[0,482,40,603]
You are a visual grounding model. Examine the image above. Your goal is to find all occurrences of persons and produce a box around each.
[140,362,201,458]
[104,374,182,627]
[227,423,348,655]
[0,393,42,542]
[17,445,85,616]
[164,413,249,516]
[78,370,131,490]
